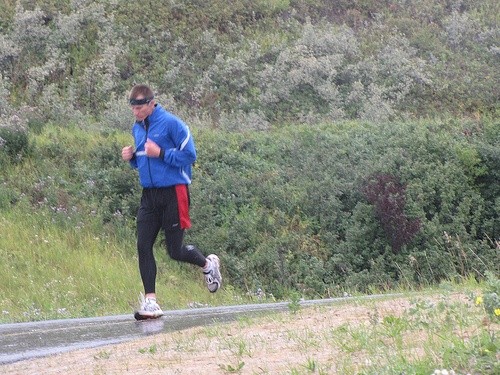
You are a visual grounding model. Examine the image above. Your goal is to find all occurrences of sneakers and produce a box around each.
[134,300,163,321]
[203,254,222,293]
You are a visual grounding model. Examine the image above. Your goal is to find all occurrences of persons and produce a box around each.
[120,84,223,321]
